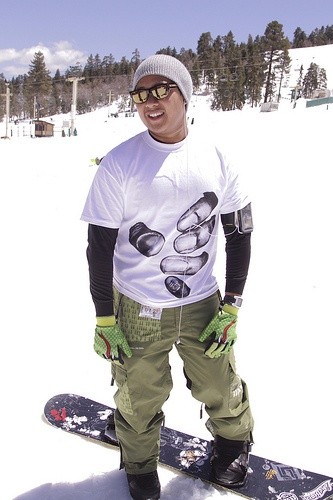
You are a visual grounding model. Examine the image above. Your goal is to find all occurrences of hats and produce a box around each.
[132,54,194,106]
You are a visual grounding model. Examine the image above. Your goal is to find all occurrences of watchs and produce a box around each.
[224,295,244,309]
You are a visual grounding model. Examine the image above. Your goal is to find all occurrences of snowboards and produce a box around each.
[43,392,333,500]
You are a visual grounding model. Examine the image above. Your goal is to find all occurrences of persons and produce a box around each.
[78,55,255,500]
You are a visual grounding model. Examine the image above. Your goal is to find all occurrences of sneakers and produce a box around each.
[213,434,253,487]
[128,471,161,500]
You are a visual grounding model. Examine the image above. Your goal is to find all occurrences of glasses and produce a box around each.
[128,82,178,105]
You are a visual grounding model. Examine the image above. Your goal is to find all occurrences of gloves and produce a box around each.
[199,301,239,359]
[93,315,133,367]
